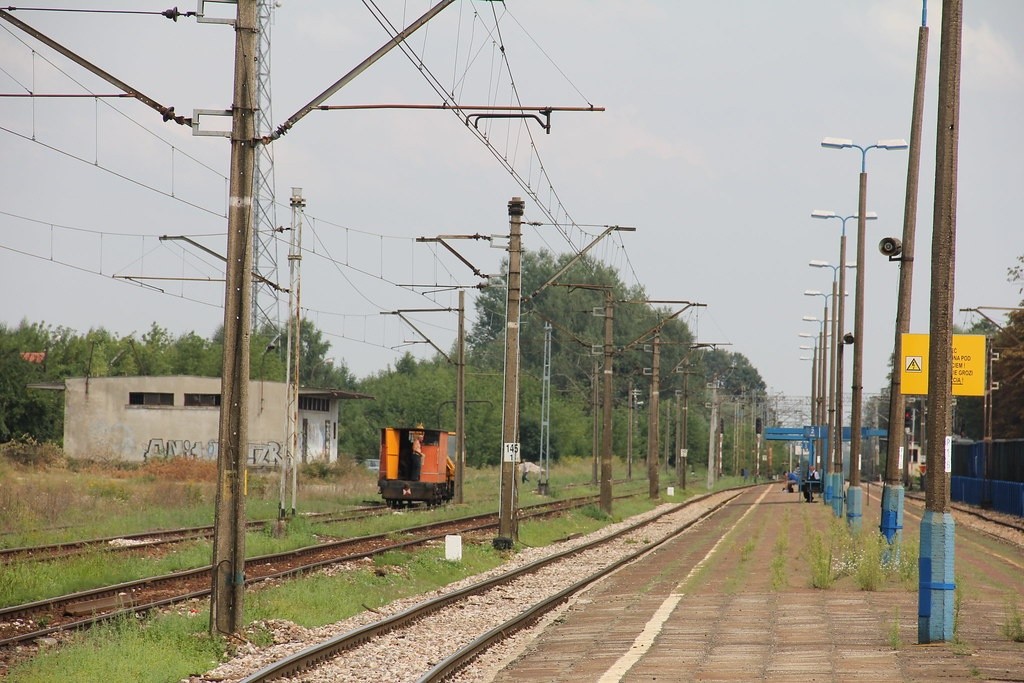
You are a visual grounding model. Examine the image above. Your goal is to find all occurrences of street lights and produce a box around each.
[797,139,906,534]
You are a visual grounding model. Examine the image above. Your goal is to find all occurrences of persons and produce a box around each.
[783,471,798,492]
[803,464,820,502]
[411,435,424,481]
[522,458,530,484]
[795,462,799,473]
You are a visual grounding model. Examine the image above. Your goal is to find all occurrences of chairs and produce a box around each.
[804,468,823,503]
